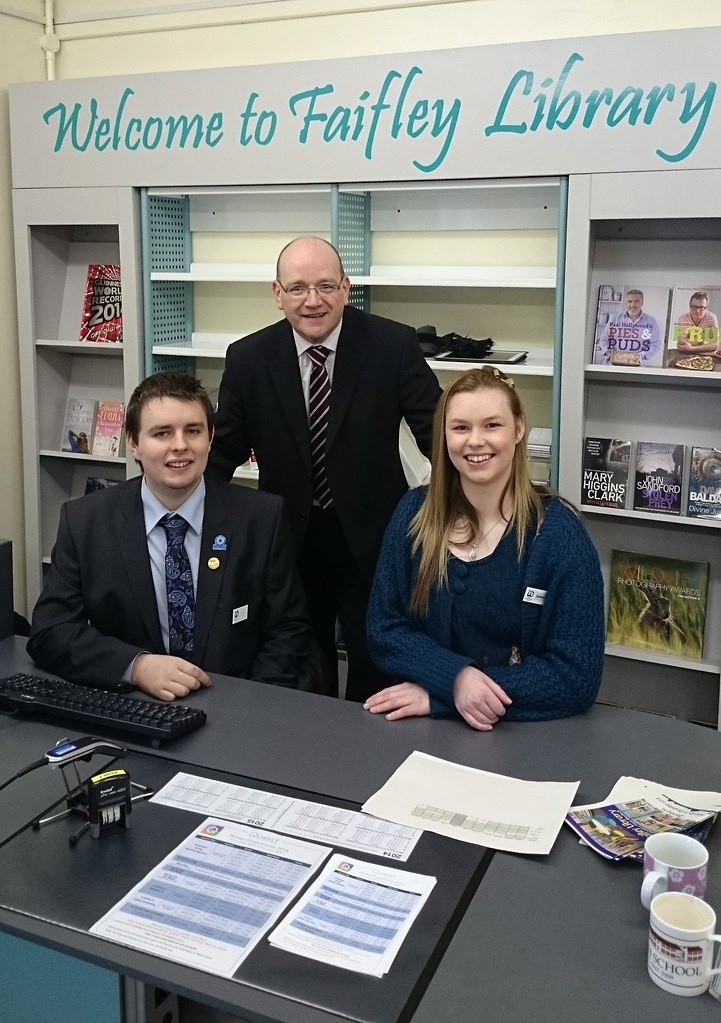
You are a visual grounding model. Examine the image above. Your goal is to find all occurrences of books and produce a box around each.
[580,436,721,525]
[591,283,721,372]
[605,549,710,660]
[62,397,127,458]
[79,264,123,342]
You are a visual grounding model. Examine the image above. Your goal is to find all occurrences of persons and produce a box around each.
[67,431,89,455]
[200,236,446,703]
[362,365,606,729]
[675,292,721,358]
[599,289,661,368]
[25,370,339,702]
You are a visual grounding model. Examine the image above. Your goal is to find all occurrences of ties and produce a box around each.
[157,513,195,663]
[305,344,334,511]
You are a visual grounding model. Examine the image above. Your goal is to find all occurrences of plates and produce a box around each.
[713,349,721,358]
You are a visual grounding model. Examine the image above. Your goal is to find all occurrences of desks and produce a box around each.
[0,636,721,1023]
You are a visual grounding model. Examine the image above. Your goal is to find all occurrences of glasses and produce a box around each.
[690,305,707,311]
[278,276,344,297]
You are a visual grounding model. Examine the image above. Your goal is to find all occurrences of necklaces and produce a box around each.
[461,500,514,561]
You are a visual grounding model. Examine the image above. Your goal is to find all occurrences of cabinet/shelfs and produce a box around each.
[12,167,721,732]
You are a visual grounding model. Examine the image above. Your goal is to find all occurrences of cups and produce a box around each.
[640,833,709,912]
[647,892,721,997]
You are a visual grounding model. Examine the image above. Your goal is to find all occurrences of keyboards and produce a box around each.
[0,672,208,739]
